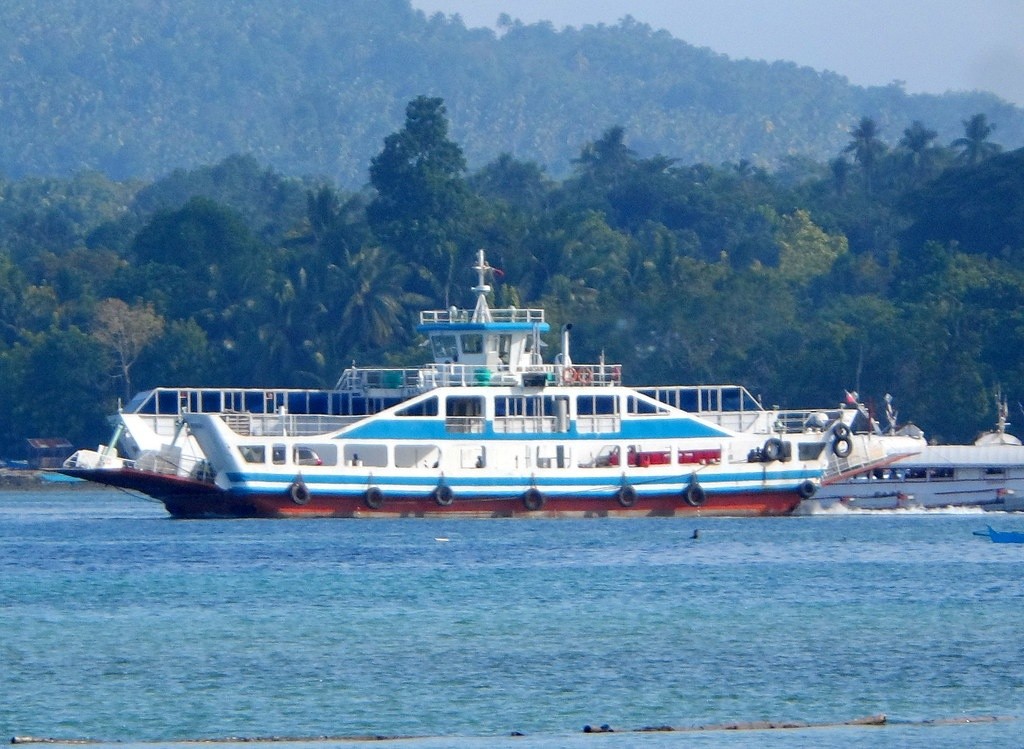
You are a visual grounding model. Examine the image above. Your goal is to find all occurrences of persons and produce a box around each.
[890,468,897,479]
[353,454,358,466]
[994,491,1006,504]
[905,469,911,479]
[476,456,482,468]
[497,356,502,371]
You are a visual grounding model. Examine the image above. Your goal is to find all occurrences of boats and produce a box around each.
[39,472,87,483]
[803,384,1024,510]
[180,323,859,522]
[39,247,928,521]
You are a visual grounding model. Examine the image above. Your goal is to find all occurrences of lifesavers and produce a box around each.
[524,488,543,511]
[562,367,593,384]
[434,486,455,506]
[365,486,384,509]
[763,438,784,460]
[799,480,816,499]
[289,481,311,505]
[684,483,706,507]
[618,485,636,507]
[833,422,853,458]
